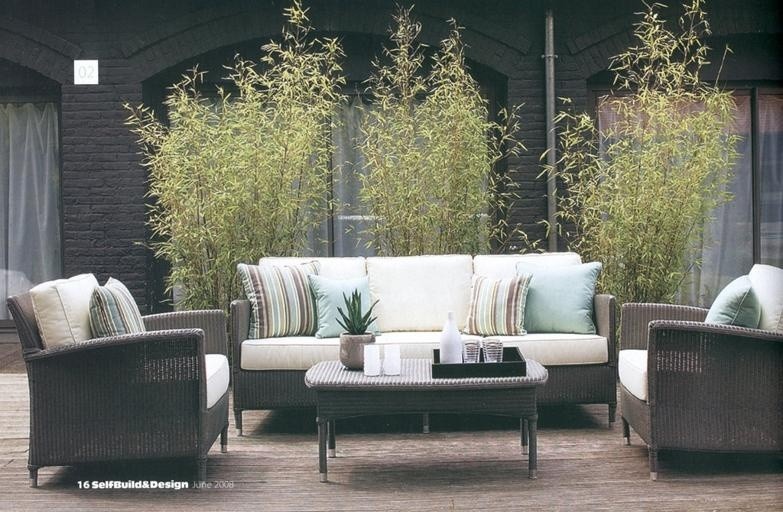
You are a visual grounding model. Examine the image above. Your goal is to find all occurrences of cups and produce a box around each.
[462,338,504,363]
[363,344,401,376]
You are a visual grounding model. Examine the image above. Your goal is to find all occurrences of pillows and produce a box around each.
[703,275,762,328]
[237,261,322,339]
[748,263,783,334]
[474,251,584,278]
[463,272,534,336]
[514,261,603,336]
[29,273,100,350]
[88,277,148,339]
[367,255,472,333]
[259,257,367,275]
[308,274,377,338]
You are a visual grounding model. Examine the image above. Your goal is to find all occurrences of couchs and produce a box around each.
[230,253,616,436]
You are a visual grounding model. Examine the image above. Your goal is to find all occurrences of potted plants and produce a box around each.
[335,287,381,369]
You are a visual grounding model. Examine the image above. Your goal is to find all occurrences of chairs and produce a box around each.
[620,263,783,483]
[6,273,231,486]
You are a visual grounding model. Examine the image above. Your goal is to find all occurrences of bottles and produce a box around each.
[440,311,463,364]
